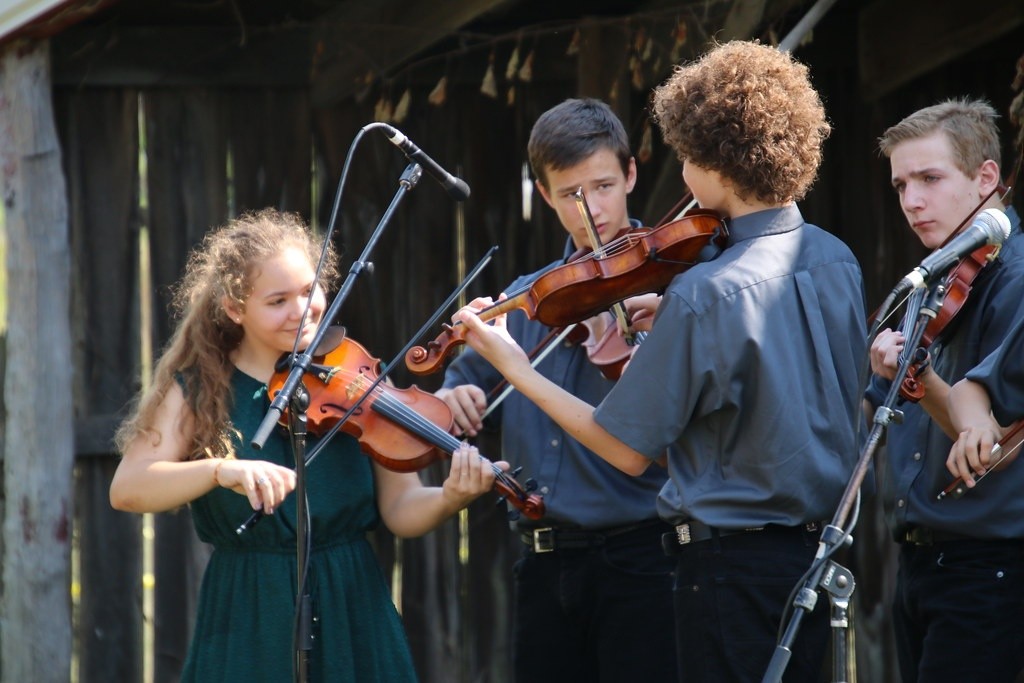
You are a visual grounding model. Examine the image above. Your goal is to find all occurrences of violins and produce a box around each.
[402,207,724,380]
[562,226,667,383]
[895,210,1012,402]
[266,324,546,527]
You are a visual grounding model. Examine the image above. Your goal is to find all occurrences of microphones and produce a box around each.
[890,208,1012,296]
[379,123,471,200]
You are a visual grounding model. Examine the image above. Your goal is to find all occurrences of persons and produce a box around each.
[862,94,1024,682]
[432,96,679,683]
[108,209,511,683]
[450,41,879,683]
